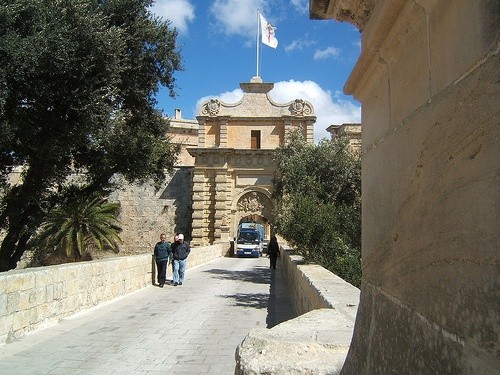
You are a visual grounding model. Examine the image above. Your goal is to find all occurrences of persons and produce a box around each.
[268,236,281,270]
[168,234,179,284]
[171,234,191,286]
[154,233,171,287]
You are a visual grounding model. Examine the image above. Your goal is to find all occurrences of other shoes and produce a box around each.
[174,282,178,286]
[159,283,164,287]
[179,282,182,285]
[270,266,276,270]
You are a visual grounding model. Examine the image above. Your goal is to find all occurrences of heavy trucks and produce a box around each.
[234,222,264,257]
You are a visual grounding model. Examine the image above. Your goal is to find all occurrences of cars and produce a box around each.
[262,239,269,249]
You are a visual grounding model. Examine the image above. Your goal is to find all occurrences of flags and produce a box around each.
[259,13,278,49]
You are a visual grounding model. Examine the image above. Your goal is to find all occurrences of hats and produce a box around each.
[178,234,184,240]
